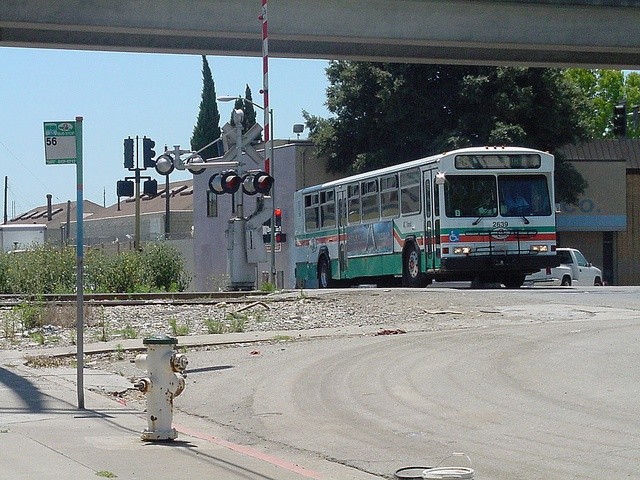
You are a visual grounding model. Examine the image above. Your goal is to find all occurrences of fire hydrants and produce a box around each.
[131,336,189,440]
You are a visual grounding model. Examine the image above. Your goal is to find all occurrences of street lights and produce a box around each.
[217,96,275,288]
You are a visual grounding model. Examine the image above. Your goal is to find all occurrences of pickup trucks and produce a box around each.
[524,248,602,286]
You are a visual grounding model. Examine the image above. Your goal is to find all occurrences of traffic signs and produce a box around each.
[43,122,76,164]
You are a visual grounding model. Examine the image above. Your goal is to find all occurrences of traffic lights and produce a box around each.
[143,139,156,167]
[276,234,286,242]
[613,106,626,137]
[117,181,134,196]
[276,209,281,226]
[222,171,272,194]
[143,179,157,196]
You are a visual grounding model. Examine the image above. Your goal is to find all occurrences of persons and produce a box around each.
[504,187,528,213]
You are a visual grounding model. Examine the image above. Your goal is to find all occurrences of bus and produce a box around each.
[296,146,560,289]
